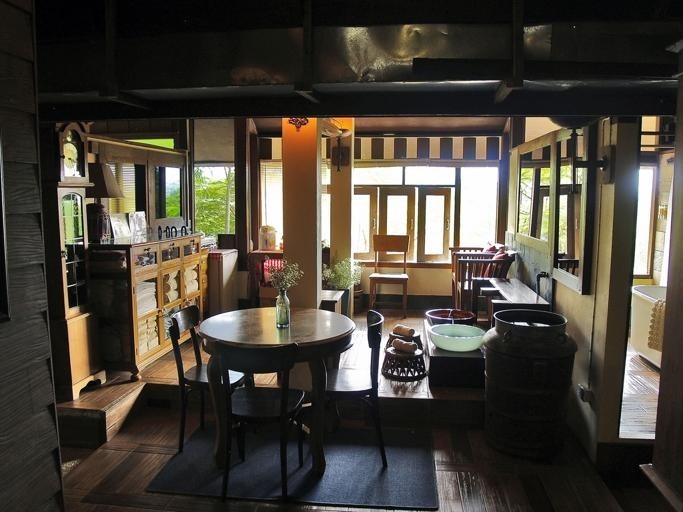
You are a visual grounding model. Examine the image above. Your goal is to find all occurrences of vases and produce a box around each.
[276,288,291,327]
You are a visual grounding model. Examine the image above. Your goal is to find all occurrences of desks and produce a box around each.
[423,318,485,387]
[197,306,356,478]
[248,250,329,310]
[472,275,550,328]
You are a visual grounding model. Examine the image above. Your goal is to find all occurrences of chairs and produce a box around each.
[449,245,506,315]
[288,310,388,470]
[168,304,247,455]
[205,340,306,508]
[456,250,518,322]
[369,235,409,319]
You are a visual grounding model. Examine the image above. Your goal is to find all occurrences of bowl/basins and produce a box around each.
[425,309,476,326]
[427,324,485,352]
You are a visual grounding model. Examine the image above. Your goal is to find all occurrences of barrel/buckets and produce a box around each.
[480,307,578,460]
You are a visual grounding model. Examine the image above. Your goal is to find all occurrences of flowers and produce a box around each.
[268,254,306,290]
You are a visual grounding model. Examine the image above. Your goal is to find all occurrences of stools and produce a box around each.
[380,346,426,381]
[384,331,424,353]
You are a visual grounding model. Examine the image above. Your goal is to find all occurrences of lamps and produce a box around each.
[86,163,125,244]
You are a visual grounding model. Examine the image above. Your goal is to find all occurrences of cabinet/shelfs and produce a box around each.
[208,248,238,316]
[93,233,203,383]
[57,182,106,401]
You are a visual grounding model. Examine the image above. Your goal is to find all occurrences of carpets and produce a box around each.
[146,420,439,509]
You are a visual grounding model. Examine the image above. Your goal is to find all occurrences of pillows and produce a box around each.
[479,242,494,252]
[485,249,507,278]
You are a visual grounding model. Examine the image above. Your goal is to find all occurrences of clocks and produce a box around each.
[58,121,89,182]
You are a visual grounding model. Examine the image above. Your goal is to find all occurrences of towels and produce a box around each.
[391,337,417,352]
[393,324,415,335]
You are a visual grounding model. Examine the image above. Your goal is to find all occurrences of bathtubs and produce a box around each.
[632,284,665,372]
